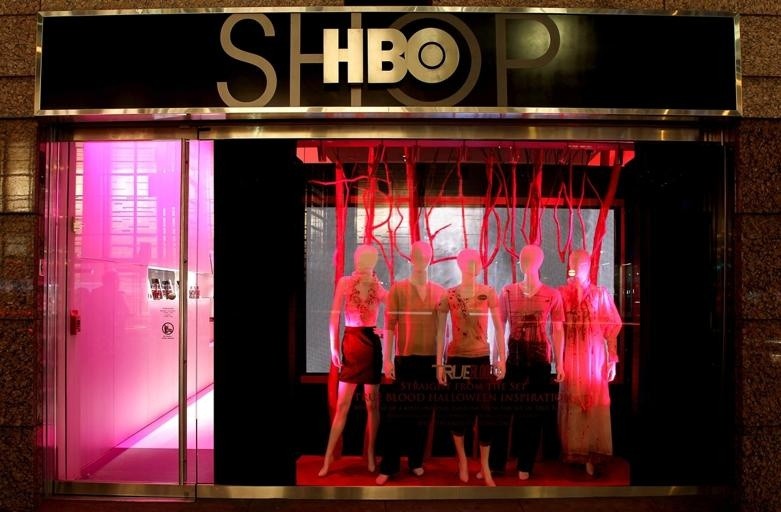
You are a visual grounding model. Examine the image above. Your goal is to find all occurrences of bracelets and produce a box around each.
[437,364,445,368]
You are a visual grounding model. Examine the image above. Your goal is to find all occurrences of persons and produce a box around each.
[374,241,446,484]
[550,249,622,476]
[318,245,389,477]
[477,247,566,480]
[435,250,508,486]
